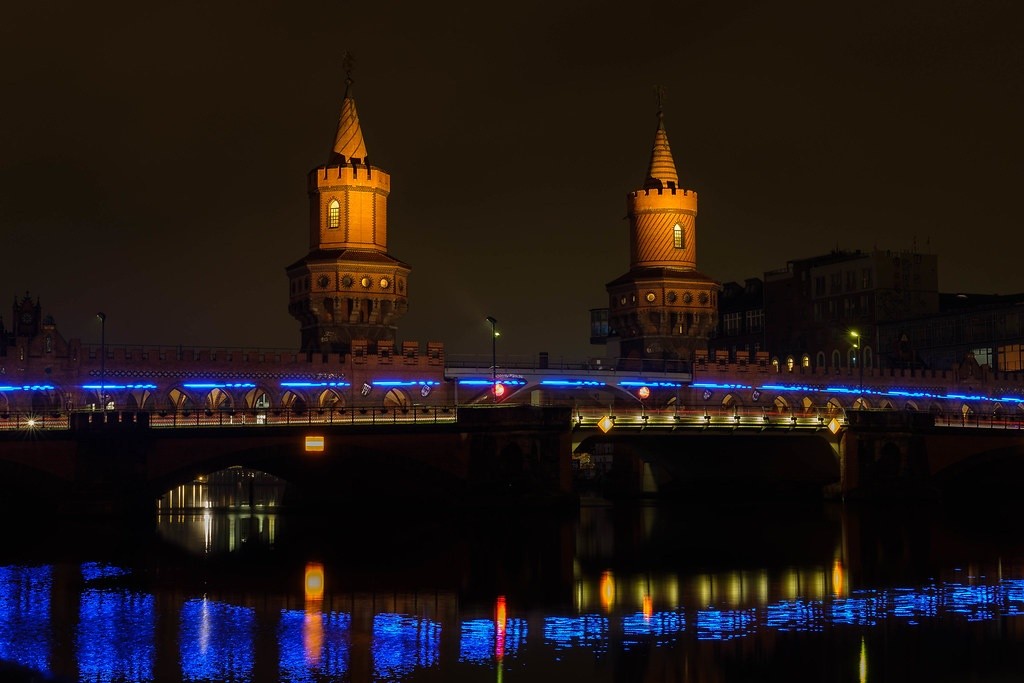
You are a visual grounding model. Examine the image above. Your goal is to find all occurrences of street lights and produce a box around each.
[485,317,498,380]
[847,327,864,392]
[96,311,107,410]
